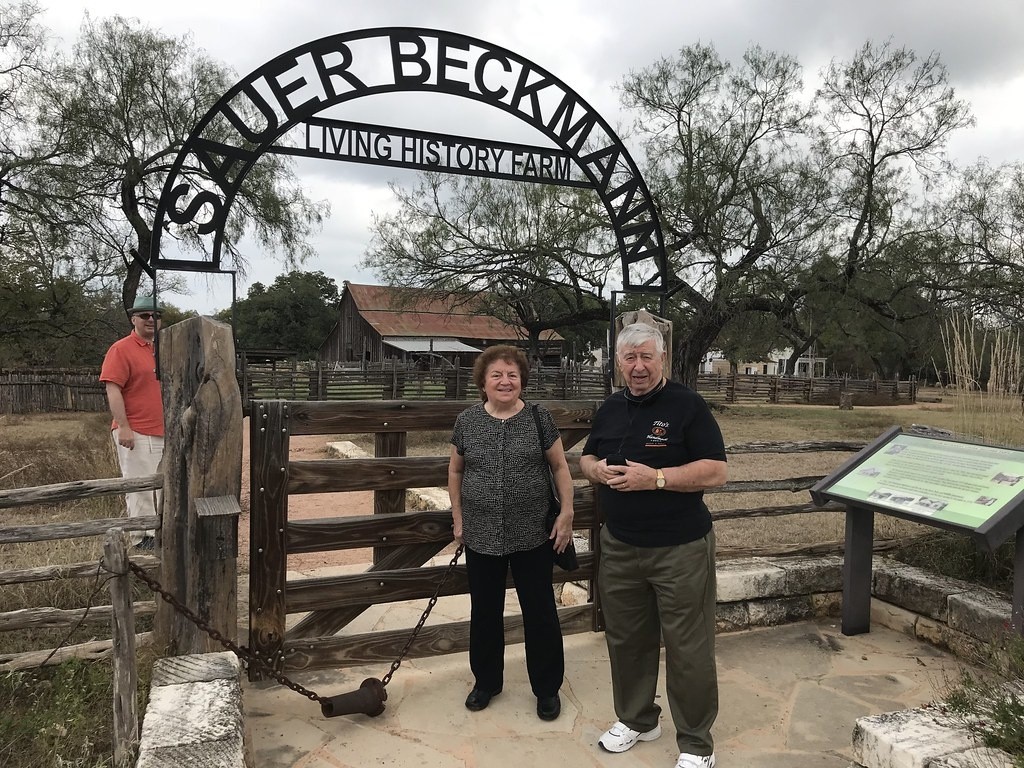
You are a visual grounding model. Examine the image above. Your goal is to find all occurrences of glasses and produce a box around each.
[133,313,162,320]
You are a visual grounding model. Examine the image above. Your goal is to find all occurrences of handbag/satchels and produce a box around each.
[545,500,580,572]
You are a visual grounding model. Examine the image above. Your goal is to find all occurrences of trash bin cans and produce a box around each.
[838,392,854,409]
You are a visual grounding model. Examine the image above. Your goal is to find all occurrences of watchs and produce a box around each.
[655,469,666,489]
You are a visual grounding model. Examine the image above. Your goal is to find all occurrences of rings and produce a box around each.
[623,483,625,488]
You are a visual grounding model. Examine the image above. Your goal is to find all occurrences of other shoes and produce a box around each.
[136,535,154,551]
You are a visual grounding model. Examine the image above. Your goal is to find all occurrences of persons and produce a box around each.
[448,344,574,721]
[100,296,166,550]
[579,323,728,768]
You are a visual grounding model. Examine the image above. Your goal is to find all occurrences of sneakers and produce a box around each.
[465,683,503,712]
[598,715,662,753]
[537,691,561,722]
[675,751,716,768]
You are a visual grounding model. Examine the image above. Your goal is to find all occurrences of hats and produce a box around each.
[127,296,166,312]
